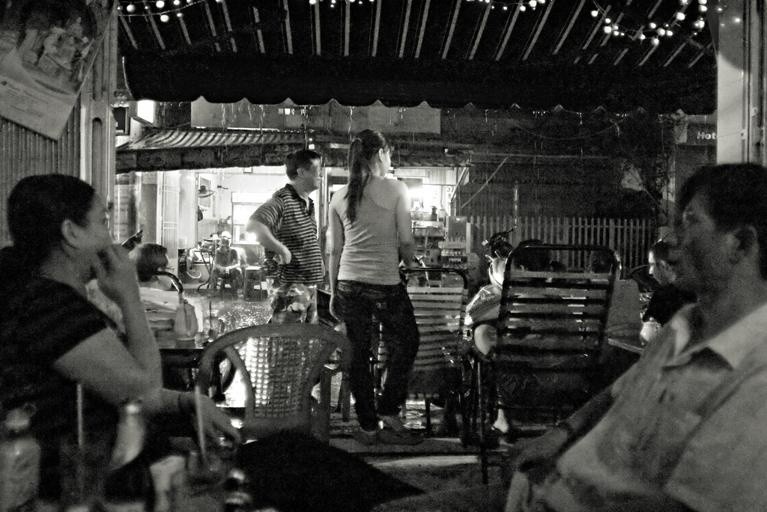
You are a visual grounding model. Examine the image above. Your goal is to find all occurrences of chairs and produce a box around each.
[190,323,356,444]
[3,365,84,506]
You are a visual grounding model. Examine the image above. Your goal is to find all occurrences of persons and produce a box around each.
[473,238,578,372]
[640,241,699,327]
[0,173,244,512]
[387,156,766,510]
[126,243,200,338]
[206,237,243,296]
[244,147,328,419]
[325,127,428,446]
[587,245,622,280]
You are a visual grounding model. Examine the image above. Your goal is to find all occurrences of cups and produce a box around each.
[173,305,197,342]
[639,316,660,342]
[201,309,219,342]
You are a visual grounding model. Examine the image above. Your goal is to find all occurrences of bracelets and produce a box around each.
[553,419,575,445]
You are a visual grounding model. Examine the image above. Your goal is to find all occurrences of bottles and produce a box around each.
[2,402,41,509]
[101,402,153,510]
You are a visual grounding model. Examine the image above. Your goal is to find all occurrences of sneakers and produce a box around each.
[353,431,378,446]
[379,428,422,445]
